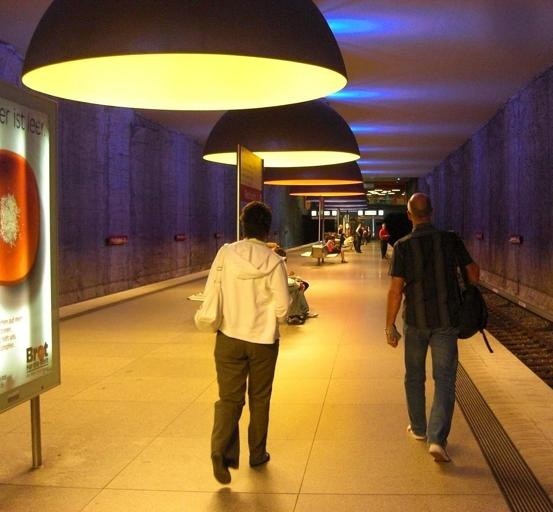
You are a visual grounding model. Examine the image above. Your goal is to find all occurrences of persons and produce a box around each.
[338,222,371,263]
[324,233,349,263]
[384,190,481,462]
[192,200,296,484]
[379,222,390,259]
[274,247,319,318]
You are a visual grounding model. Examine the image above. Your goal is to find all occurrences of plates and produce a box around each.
[0,149,40,287]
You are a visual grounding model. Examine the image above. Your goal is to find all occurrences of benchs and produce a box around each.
[188,291,206,305]
[302,243,337,265]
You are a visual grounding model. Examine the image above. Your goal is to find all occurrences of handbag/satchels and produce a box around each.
[458,285,488,340]
[193,281,223,332]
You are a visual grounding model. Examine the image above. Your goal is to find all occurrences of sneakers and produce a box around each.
[211,450,231,485]
[429,443,451,463]
[408,424,426,440]
[249,452,270,467]
[307,312,318,318]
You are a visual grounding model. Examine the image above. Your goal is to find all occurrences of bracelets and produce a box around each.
[385,325,397,335]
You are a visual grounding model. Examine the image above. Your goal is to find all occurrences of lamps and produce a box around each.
[21,0,348,112]
[202,102,369,211]
